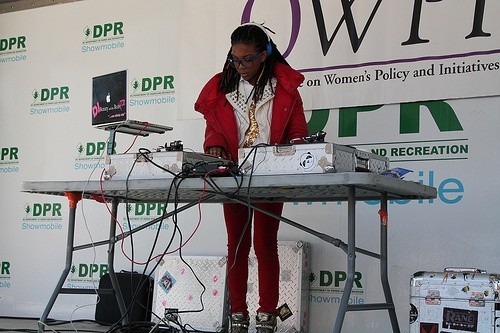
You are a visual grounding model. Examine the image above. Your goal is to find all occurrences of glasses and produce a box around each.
[227,53,262,69]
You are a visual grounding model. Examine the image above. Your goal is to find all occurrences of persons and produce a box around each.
[194,21,309,333]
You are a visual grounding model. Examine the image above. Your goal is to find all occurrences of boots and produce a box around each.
[256,311,278,333]
[230,312,250,333]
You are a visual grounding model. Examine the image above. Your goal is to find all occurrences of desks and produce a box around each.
[21,171,438,333]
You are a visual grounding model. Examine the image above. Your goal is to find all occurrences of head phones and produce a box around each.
[241,22,271,55]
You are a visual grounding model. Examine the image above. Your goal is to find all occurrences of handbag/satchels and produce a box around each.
[95,270,154,326]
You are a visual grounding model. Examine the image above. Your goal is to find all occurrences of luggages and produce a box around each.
[409,267,500,333]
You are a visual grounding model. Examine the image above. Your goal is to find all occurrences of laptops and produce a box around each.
[92,69,173,135]
